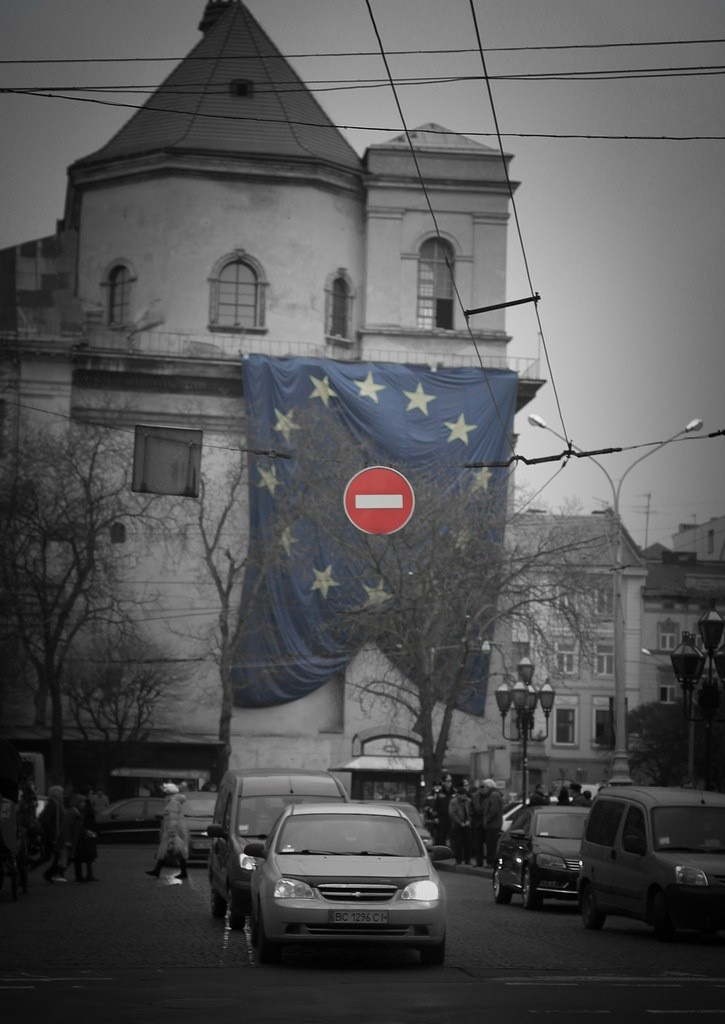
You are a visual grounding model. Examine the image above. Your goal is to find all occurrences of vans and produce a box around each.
[578,785,725,946]
[205,769,351,930]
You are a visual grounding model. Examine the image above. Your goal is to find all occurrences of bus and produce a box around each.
[18,751,46,797]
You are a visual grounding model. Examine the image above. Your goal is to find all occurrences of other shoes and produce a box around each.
[86,876,99,881]
[456,859,461,864]
[464,859,470,864]
[43,871,53,883]
[145,871,160,877]
[485,864,495,868]
[75,877,88,882]
[473,864,483,867]
[175,872,187,878]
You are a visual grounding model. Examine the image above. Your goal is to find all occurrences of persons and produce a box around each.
[427,774,502,868]
[13,780,107,893]
[704,782,716,792]
[145,782,192,880]
[557,783,606,807]
[527,783,551,807]
[669,774,705,849]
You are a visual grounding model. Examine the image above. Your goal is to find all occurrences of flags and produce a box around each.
[230,349,520,718]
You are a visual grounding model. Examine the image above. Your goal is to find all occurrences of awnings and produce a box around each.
[0,725,225,752]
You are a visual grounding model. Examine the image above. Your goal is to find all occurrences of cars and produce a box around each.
[181,789,219,861]
[357,801,434,852]
[245,802,452,966]
[98,797,166,840]
[492,806,591,906]
[500,797,573,834]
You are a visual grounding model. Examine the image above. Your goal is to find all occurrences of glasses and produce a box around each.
[480,786,484,788]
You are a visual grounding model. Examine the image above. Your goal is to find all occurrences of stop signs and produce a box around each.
[343,465,416,536]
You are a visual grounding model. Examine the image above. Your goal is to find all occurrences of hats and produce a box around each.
[163,783,180,793]
[568,784,581,792]
[442,774,453,781]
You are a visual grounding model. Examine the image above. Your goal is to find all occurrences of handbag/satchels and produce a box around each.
[57,846,70,868]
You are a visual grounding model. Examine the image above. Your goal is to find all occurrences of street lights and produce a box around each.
[642,648,702,786]
[493,658,555,803]
[528,417,703,787]
[670,602,725,788]
